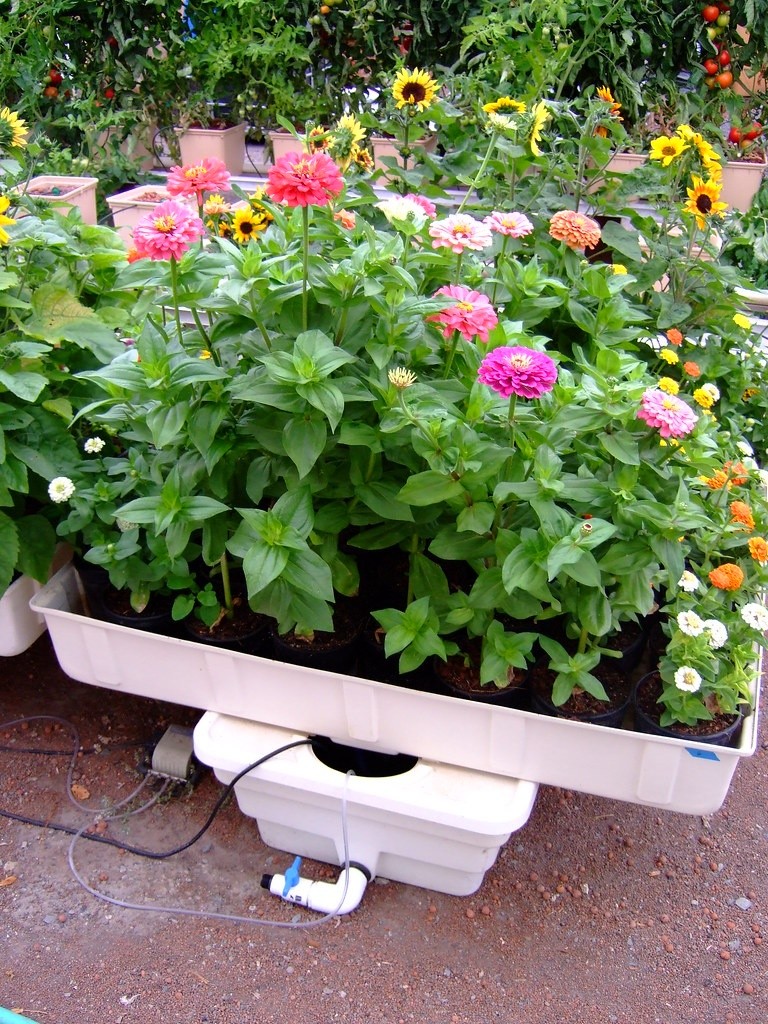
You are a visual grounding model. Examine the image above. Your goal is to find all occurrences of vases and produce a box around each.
[183,610,272,653]
[8,176,100,227]
[87,118,156,171]
[716,145,768,215]
[270,122,332,168]
[107,184,199,251]
[272,620,356,671]
[175,120,247,177]
[371,131,437,187]
[96,584,178,635]
[601,620,648,667]
[587,153,649,204]
[634,669,743,746]
[435,655,531,708]
[533,661,636,726]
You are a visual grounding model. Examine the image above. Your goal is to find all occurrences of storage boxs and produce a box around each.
[28,469,766,818]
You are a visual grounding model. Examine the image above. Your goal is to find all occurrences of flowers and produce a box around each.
[1,105,768,727]
